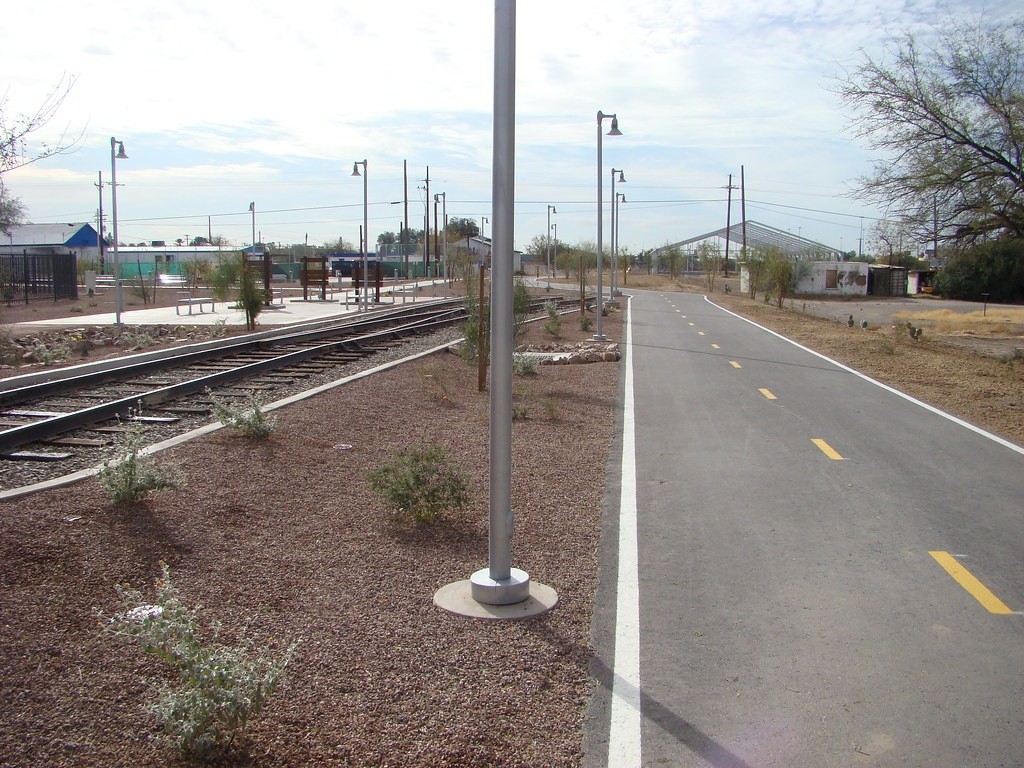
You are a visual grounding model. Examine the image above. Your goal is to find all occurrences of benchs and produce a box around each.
[346,288,374,311]
[176,291,216,315]
[393,284,416,303]
[272,291,284,304]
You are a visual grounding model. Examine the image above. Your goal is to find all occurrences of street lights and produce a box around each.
[615,192,627,291]
[111,137,130,324]
[551,224,556,269]
[597,111,624,336]
[482,217,489,264]
[611,168,627,301]
[249,202,255,260]
[351,159,367,313]
[548,205,557,287]
[434,192,446,299]
